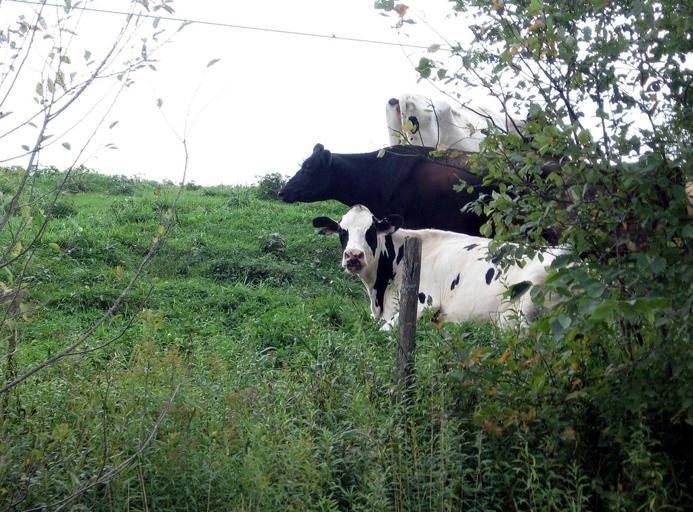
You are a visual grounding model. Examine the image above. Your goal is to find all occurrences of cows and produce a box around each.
[311,204,624,339]
[386,92,530,153]
[279,143,555,264]
